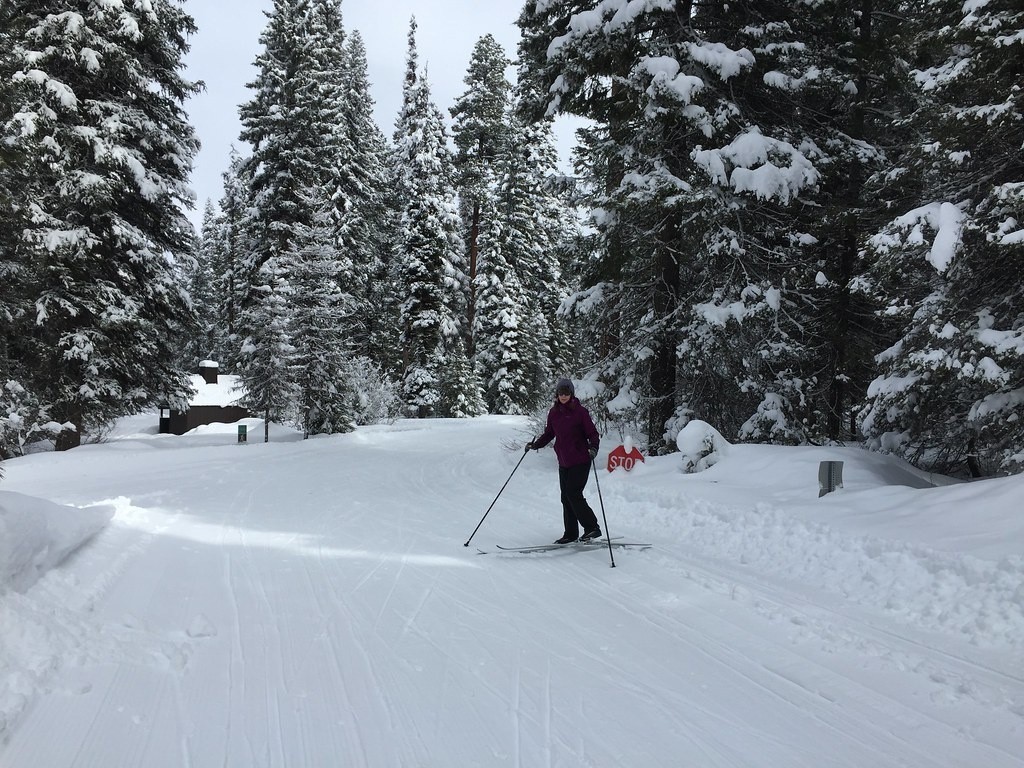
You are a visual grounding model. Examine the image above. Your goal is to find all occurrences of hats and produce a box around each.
[556,379,574,396]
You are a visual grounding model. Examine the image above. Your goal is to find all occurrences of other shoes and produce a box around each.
[554,537,578,544]
[579,524,602,542]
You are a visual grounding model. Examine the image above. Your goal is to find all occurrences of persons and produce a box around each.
[525,378,601,543]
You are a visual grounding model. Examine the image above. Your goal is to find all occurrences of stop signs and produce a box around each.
[608,445,645,473]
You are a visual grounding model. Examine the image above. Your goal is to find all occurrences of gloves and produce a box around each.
[525,442,538,452]
[589,447,597,460]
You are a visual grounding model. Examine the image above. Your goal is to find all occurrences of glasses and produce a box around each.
[557,387,570,396]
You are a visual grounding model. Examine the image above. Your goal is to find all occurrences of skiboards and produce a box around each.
[476,535,653,555]
